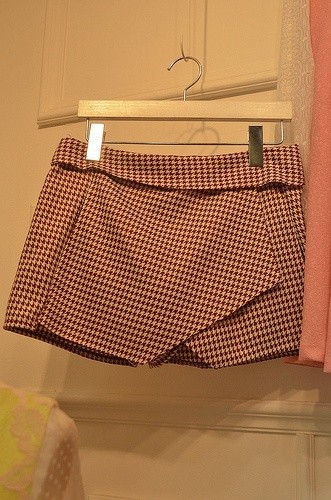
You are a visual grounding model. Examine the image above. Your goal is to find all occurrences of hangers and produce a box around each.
[75,57,294,167]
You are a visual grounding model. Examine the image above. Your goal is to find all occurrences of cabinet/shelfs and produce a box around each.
[35,0,281,132]
